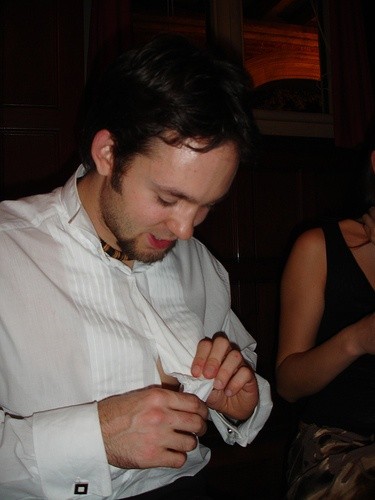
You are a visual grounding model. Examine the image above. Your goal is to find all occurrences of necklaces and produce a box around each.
[96,234,136,261]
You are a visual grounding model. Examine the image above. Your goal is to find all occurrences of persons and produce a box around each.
[273,147,375,500]
[1,49,273,499]
